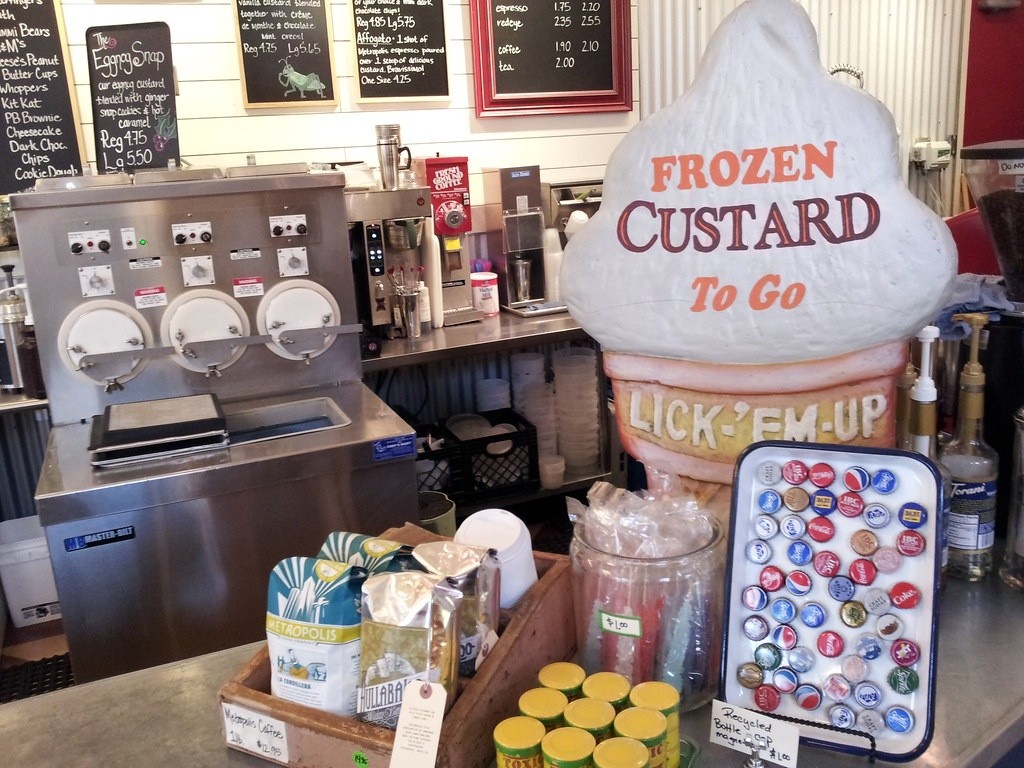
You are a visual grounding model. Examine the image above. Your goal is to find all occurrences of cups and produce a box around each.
[546,210,589,300]
[474,347,599,489]
[378,139,411,189]
[391,293,422,339]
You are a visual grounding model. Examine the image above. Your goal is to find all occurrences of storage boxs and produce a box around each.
[218,546,575,768]
[433,408,543,506]
[415,420,467,508]
[0,514,64,629]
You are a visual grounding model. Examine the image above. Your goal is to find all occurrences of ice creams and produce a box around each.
[559,0,959,550]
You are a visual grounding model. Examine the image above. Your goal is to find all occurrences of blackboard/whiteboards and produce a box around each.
[231,0,340,109]
[349,0,452,103]
[0,0,93,203]
[487,0,618,100]
[85,22,181,175]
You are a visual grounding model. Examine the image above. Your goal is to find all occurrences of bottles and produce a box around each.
[416,281,432,333]
[568,508,724,715]
